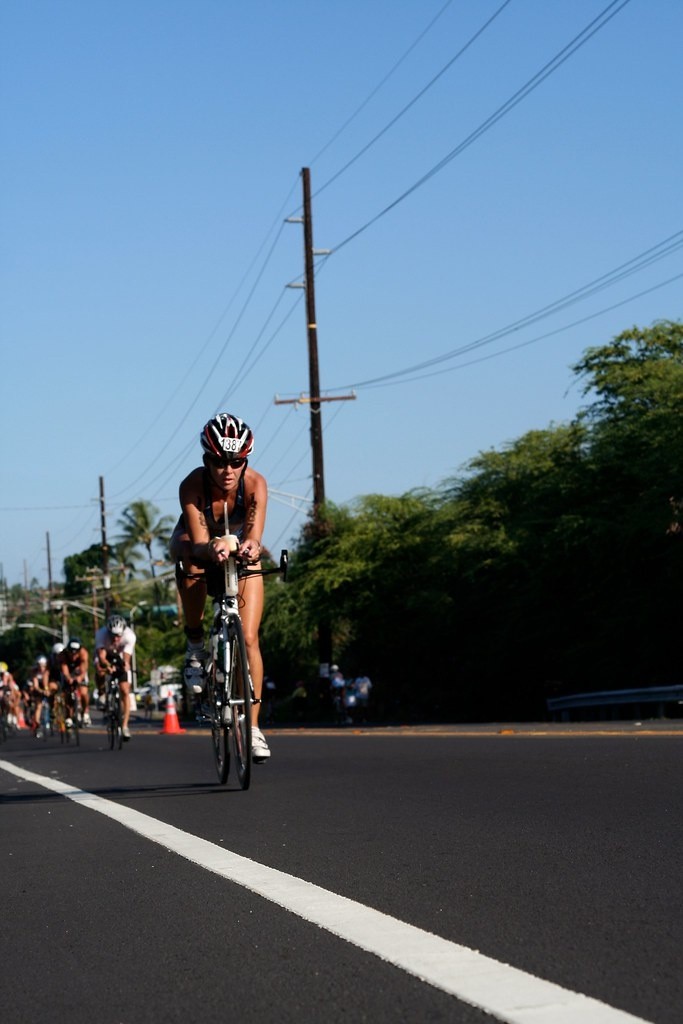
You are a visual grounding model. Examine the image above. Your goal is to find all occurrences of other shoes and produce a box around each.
[81,713,89,723]
[122,726,131,740]
[97,692,107,708]
[65,718,73,728]
[7,713,12,724]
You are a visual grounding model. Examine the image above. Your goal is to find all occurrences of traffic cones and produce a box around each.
[161,692,186,735]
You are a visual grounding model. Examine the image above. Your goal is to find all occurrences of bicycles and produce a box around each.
[163,549,288,791]
[0,661,131,751]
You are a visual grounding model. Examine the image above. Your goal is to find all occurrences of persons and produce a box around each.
[144,692,152,719]
[57,639,92,728]
[290,664,373,724]
[0,643,66,738]
[171,412,271,761]
[94,615,136,741]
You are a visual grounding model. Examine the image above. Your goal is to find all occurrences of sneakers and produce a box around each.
[249,725,272,759]
[183,641,212,694]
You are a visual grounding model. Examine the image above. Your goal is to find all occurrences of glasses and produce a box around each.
[109,632,123,637]
[206,452,246,469]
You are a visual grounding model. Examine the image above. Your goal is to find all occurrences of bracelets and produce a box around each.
[258,542,261,549]
[208,537,220,556]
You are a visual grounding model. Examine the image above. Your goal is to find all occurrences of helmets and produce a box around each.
[36,655,47,665]
[331,664,340,671]
[0,661,8,672]
[67,638,82,654]
[200,413,254,459]
[52,642,64,653]
[105,614,126,634]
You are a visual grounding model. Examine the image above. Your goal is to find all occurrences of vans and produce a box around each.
[135,664,185,713]
